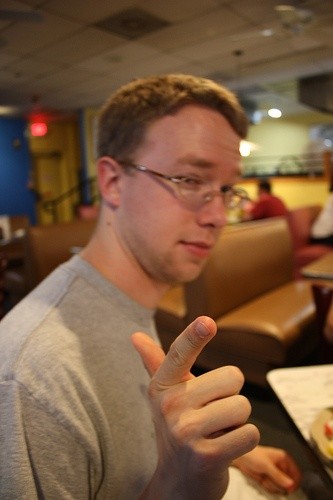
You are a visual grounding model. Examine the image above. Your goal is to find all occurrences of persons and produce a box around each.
[0,75,302,500]
[244,178,288,221]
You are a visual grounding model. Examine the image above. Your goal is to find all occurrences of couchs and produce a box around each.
[4,216,98,294]
[151,214,333,386]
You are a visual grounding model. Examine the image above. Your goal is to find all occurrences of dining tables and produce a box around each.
[266,360,333,480]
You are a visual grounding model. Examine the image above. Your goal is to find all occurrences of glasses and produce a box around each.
[115,158,249,210]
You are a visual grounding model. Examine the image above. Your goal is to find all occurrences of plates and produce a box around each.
[310,405,333,462]
